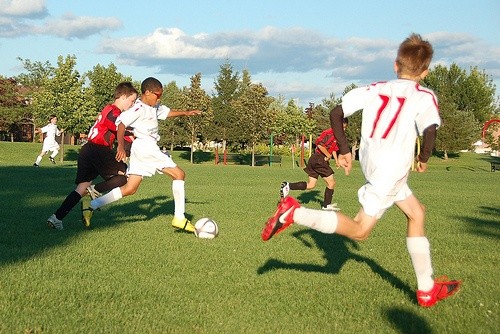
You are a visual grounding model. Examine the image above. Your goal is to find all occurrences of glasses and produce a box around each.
[153,92,162,99]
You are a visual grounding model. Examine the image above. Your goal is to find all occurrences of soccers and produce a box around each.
[193,216,220,241]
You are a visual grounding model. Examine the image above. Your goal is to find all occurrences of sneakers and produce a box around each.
[279,182,289,198]
[416,278,461,308]
[86,184,103,211]
[81,200,93,227]
[321,203,340,212]
[261,195,302,240]
[47,213,64,230]
[171,216,196,232]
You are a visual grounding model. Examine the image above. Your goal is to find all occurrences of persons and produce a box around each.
[279,116,349,211]
[47,81,138,230]
[33,114,64,167]
[263,33,461,308]
[82,76,203,233]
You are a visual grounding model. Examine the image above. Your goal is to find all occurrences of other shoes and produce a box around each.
[33,163,39,167]
[48,156,56,165]
